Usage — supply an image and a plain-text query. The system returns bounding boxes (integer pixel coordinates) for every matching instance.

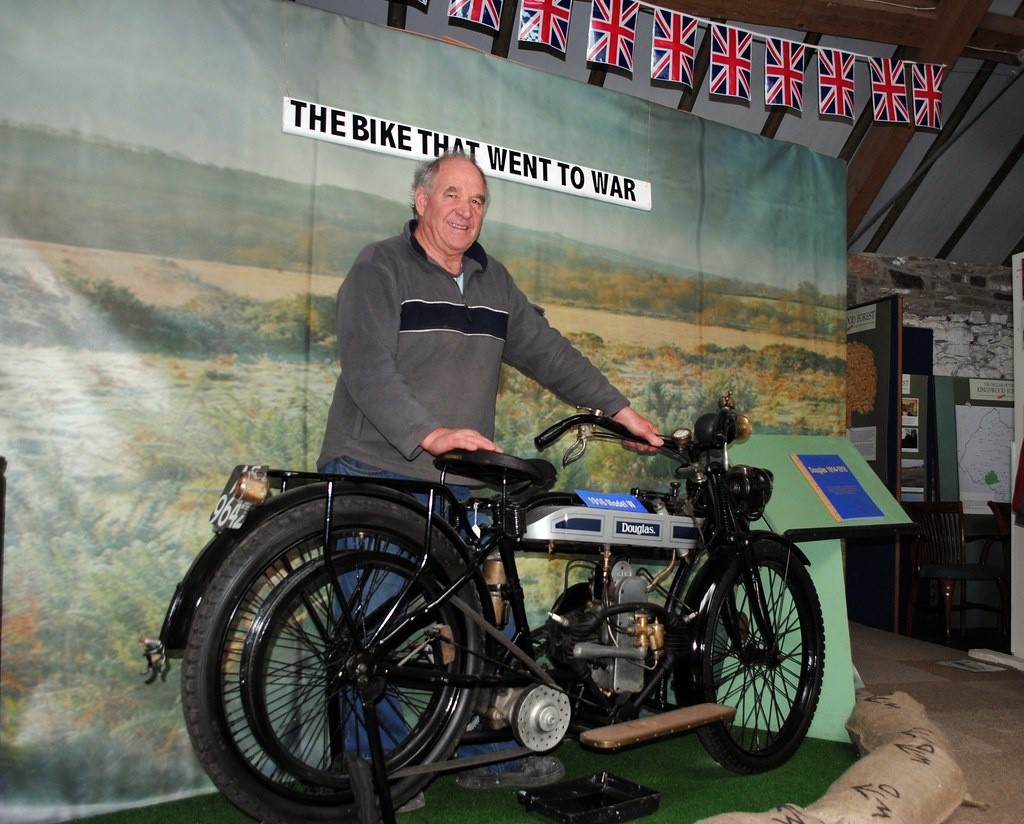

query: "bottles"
[483,549,509,626]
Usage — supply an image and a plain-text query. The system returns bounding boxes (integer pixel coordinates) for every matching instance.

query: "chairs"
[899,500,1007,647]
[988,501,1011,656]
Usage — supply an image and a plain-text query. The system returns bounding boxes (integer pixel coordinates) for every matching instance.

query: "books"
[790,454,885,523]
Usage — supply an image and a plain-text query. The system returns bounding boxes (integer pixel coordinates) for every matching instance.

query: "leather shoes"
[376,789,426,812]
[458,752,566,789]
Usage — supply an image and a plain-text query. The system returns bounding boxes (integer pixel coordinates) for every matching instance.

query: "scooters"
[139,379,828,824]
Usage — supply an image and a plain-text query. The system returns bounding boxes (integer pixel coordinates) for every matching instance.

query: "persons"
[315,153,664,814]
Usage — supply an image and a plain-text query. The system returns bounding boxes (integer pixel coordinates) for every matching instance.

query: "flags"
[709,23,752,103]
[764,37,805,113]
[817,47,856,120]
[650,8,697,89]
[868,56,910,123]
[912,63,946,130]
[517,0,573,53]
[586,0,640,72]
